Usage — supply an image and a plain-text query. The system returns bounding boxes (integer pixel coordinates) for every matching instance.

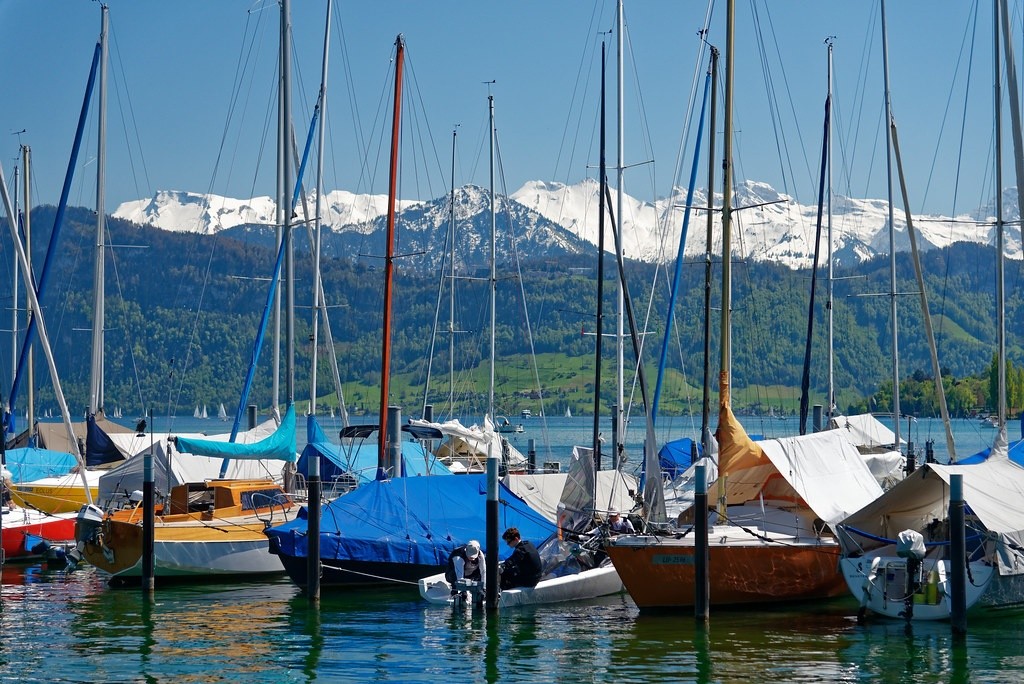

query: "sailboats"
[587,0,903,620]
[840,0,1022,617]
[0,0,538,587]
[262,30,692,615]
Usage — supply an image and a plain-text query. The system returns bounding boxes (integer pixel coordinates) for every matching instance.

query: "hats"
[608,507,620,516]
[466,540,481,559]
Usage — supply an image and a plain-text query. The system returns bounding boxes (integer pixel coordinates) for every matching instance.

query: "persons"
[500,527,542,588]
[444,539,486,595]
[584,506,635,568]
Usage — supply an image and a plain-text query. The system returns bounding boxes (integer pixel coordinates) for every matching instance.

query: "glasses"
[507,538,514,545]
